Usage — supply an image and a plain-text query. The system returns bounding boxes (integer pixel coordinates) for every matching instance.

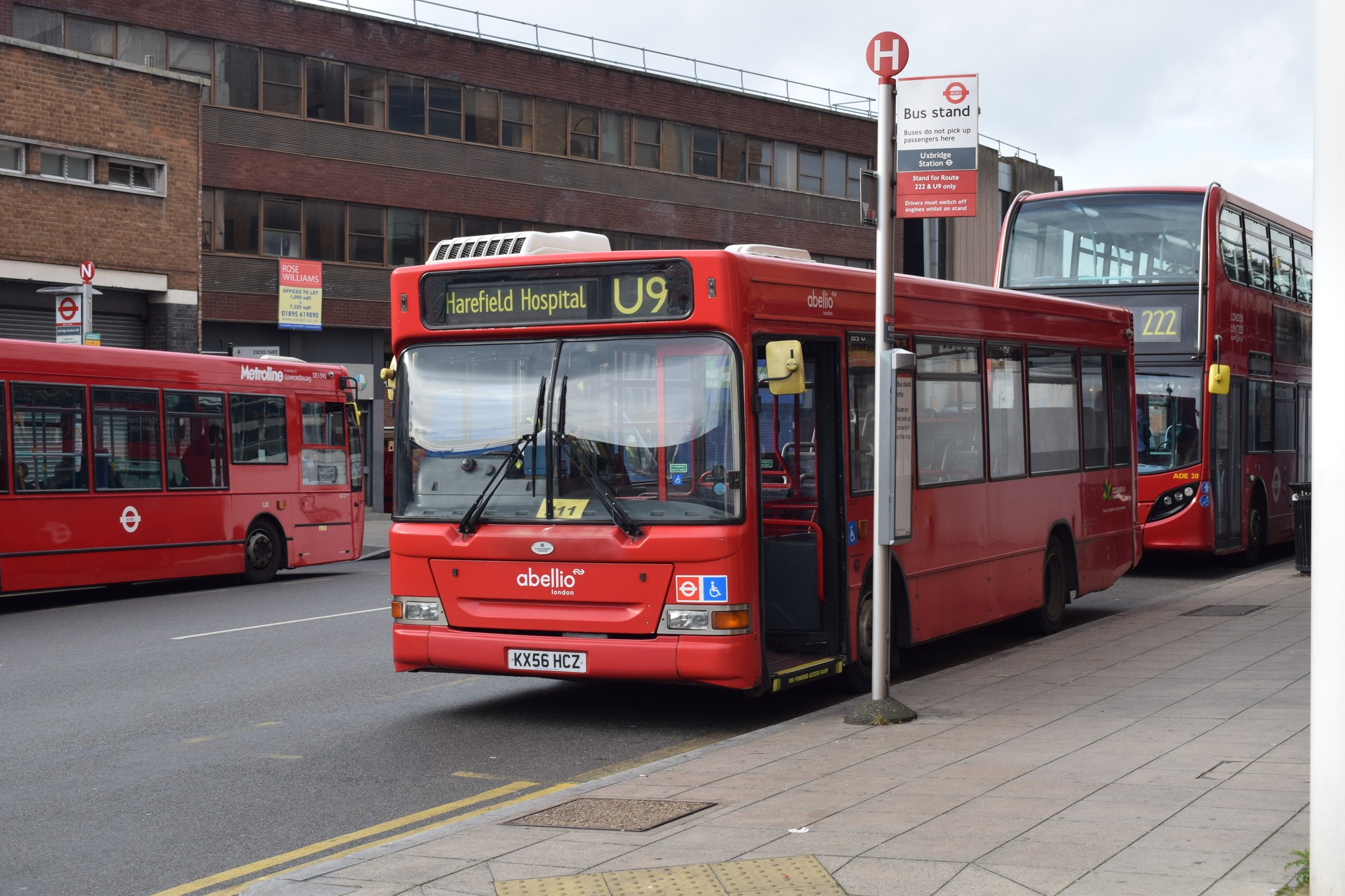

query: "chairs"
[1148,423,1197,467]
[1031,260,1313,304]
[682,404,1106,518]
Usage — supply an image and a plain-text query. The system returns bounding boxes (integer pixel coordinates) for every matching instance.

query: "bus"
[0,183,1312,717]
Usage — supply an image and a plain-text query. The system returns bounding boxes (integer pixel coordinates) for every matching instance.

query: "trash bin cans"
[1287,481,1312,577]
